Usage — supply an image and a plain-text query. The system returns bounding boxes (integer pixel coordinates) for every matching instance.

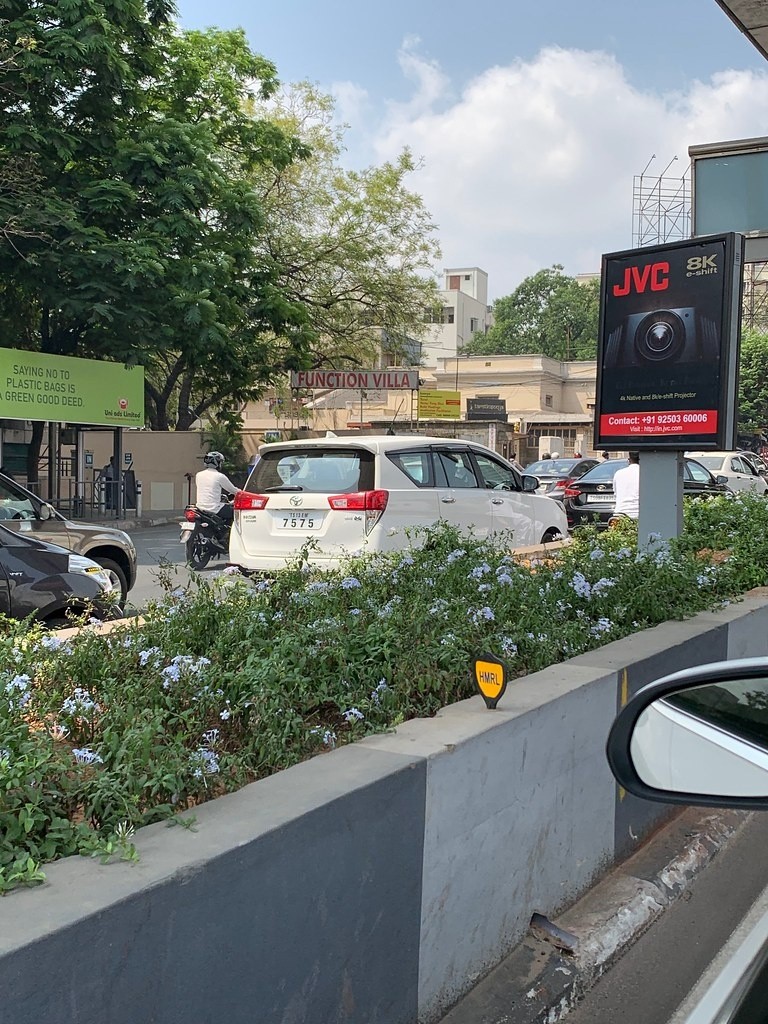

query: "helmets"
[203,452,225,471]
[543,453,550,460]
[550,451,560,460]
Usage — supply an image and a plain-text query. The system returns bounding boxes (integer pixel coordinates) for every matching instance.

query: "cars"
[561,456,736,541]
[683,449,768,503]
[731,449,768,488]
[0,522,115,637]
[517,458,602,527]
[403,459,513,491]
[603,655,768,1024]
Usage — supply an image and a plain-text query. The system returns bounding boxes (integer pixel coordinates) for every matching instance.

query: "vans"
[229,430,569,581]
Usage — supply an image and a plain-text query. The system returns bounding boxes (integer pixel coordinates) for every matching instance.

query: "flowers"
[0,491,768,891]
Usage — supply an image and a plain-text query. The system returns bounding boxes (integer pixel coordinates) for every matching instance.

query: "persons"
[508,453,526,473]
[542,453,551,460]
[195,452,243,553]
[550,452,559,460]
[0,466,16,482]
[612,450,640,523]
[602,452,610,459]
[435,454,468,487]
[573,452,582,458]
[101,456,116,510]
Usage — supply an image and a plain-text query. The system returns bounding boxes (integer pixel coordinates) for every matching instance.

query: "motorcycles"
[178,492,239,572]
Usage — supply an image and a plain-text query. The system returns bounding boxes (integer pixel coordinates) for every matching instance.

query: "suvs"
[0,473,137,612]
